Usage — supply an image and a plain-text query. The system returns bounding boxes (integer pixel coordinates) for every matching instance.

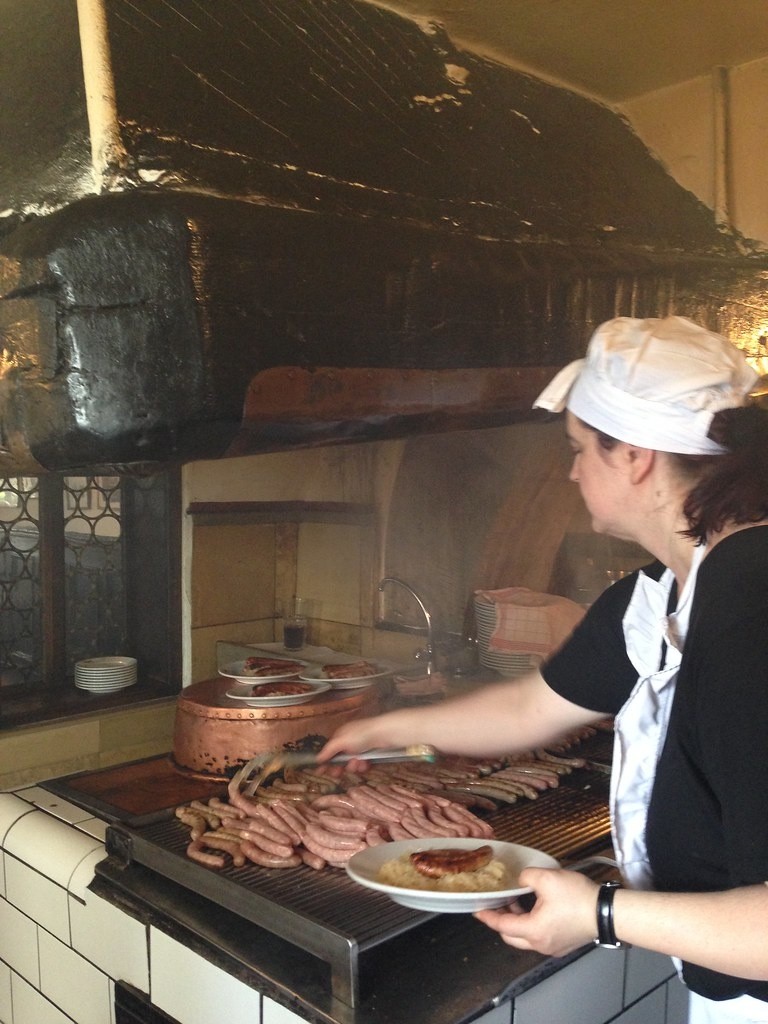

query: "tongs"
[267,743,440,770]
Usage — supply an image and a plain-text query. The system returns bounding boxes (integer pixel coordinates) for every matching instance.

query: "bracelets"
[592,879,630,954]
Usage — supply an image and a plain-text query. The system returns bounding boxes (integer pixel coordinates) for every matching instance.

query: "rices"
[381,857,506,891]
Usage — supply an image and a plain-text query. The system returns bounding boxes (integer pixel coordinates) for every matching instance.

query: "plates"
[299,667,394,689]
[474,595,536,678]
[74,656,136,693]
[226,681,332,708]
[346,837,561,913]
[218,660,302,684]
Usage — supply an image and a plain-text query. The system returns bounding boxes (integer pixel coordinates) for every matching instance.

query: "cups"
[282,598,309,651]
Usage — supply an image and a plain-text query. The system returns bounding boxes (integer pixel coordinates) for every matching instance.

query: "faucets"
[377,577,439,674]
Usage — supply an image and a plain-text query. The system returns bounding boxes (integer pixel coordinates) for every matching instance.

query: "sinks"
[373,666,494,708]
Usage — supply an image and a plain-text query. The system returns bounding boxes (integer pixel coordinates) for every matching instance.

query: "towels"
[473,587,593,667]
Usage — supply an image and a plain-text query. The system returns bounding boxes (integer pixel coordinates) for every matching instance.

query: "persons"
[322,316,768,1024]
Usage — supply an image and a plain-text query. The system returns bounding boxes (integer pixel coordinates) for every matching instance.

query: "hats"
[533,311,763,459]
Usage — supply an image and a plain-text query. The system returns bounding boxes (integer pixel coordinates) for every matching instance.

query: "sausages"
[253,681,310,694]
[177,714,613,871]
[244,656,304,676]
[323,661,376,677]
